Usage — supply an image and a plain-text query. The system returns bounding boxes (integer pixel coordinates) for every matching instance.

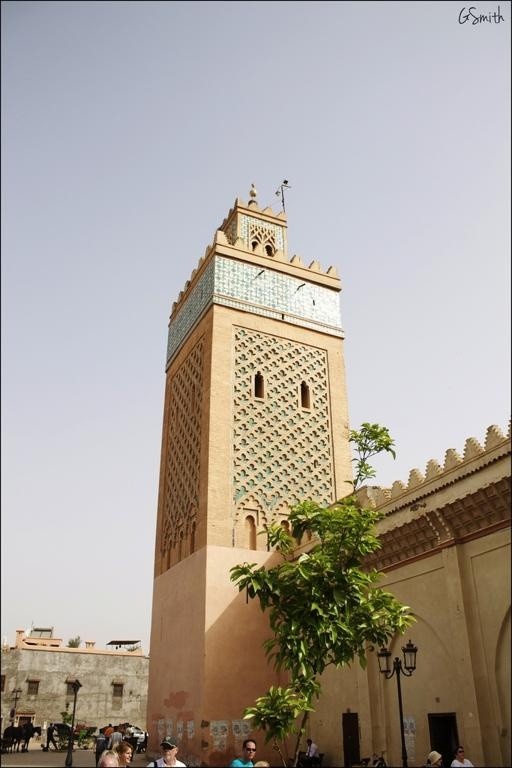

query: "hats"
[160,736,177,748]
[427,751,442,766]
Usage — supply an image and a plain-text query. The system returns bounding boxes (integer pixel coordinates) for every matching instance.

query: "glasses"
[457,750,465,753]
[162,745,173,750]
[245,745,256,752]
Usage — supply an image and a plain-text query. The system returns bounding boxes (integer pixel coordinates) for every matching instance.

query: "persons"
[228,739,256,767]
[146,735,187,767]
[73,719,149,767]
[46,722,58,751]
[424,750,443,768]
[253,759,269,767]
[302,738,321,766]
[450,746,474,767]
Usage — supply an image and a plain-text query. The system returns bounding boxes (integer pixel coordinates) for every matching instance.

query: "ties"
[308,745,310,757]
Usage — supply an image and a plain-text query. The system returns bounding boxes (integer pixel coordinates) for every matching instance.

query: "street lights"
[376,639,418,767]
[65,680,82,766]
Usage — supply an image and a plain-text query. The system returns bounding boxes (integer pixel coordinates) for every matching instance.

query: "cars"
[126,727,148,752]
